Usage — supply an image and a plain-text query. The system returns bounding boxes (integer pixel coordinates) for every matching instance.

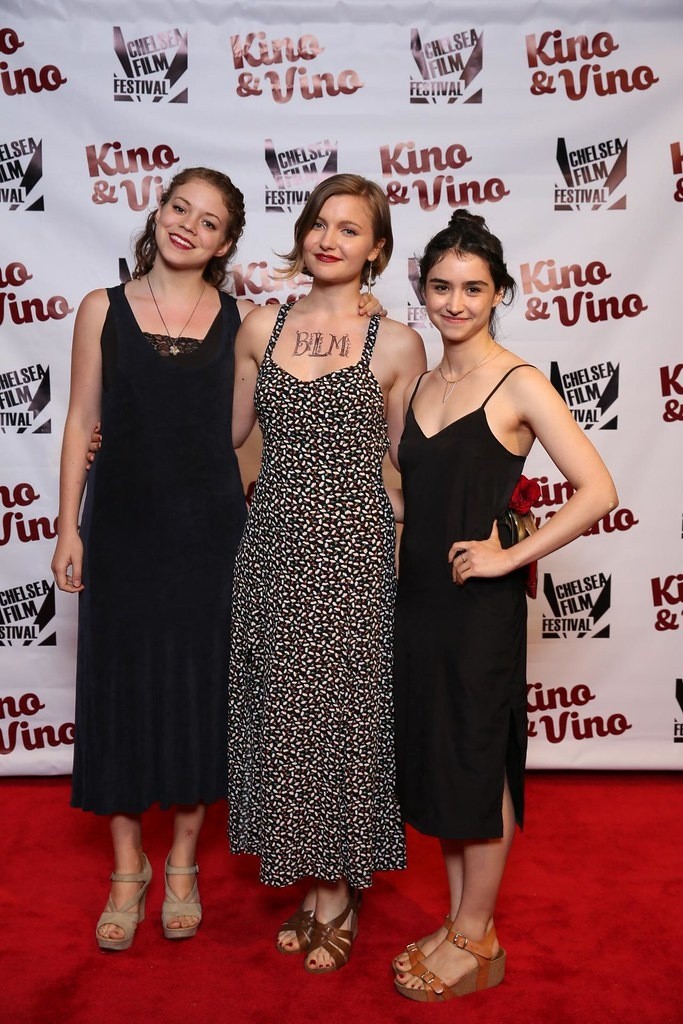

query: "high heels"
[161,848,202,939]
[275,896,316,954]
[304,888,363,974]
[394,921,506,1003]
[392,913,456,973]
[95,853,152,951]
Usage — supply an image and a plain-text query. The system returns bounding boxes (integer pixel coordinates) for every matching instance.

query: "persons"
[51,168,386,950]
[85,174,428,973]
[244,210,619,1002]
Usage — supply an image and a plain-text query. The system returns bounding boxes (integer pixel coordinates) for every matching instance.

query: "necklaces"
[438,342,508,403]
[145,275,206,355]
